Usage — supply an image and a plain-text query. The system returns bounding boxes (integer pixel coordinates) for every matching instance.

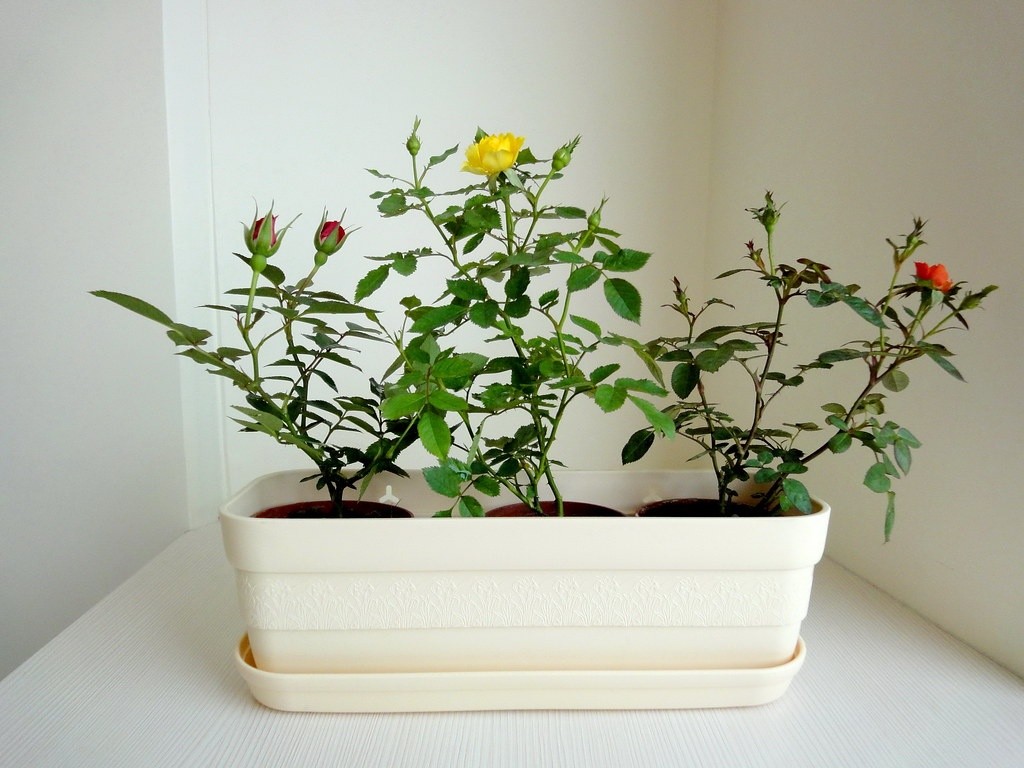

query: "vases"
[217,467,832,714]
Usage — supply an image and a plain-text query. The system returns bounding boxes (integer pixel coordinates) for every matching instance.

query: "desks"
[0,520,1024,768]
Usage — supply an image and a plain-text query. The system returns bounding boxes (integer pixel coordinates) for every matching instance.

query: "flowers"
[87,113,998,546]
